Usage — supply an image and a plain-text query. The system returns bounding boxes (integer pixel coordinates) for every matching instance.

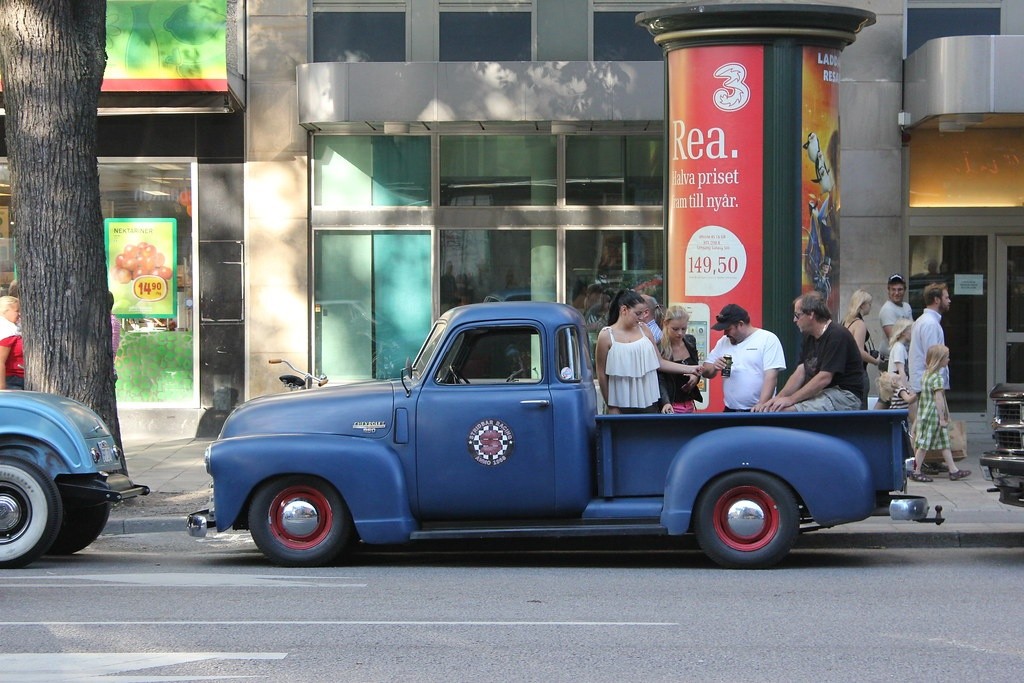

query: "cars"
[0,389,150,569]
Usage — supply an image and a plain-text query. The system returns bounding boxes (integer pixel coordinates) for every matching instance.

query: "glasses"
[716,315,739,323]
[794,311,807,319]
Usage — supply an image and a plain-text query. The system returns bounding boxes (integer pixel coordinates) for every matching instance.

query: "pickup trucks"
[187,301,945,568]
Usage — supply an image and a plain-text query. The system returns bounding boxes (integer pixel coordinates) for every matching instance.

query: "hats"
[711,304,748,331]
[887,273,906,285]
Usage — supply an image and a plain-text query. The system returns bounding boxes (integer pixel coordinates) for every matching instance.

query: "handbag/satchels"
[909,391,968,463]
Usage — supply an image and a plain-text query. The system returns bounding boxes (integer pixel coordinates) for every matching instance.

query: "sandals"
[911,473,934,482]
[950,469,972,481]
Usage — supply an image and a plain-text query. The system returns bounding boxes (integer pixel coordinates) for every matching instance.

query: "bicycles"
[268,358,329,392]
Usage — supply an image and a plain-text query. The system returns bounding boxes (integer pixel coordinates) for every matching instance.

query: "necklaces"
[820,324,826,335]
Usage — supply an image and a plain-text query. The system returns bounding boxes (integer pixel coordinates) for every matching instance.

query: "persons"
[759,295,864,412]
[0,279,24,390]
[909,284,951,471]
[877,372,938,475]
[640,295,663,342]
[910,345,971,481]
[702,304,786,412]
[655,306,703,414]
[839,290,885,410]
[874,274,913,409]
[584,284,609,361]
[595,289,704,414]
[109,292,121,381]
[888,319,913,376]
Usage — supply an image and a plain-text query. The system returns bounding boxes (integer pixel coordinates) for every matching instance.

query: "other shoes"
[932,461,949,472]
[920,463,938,474]
